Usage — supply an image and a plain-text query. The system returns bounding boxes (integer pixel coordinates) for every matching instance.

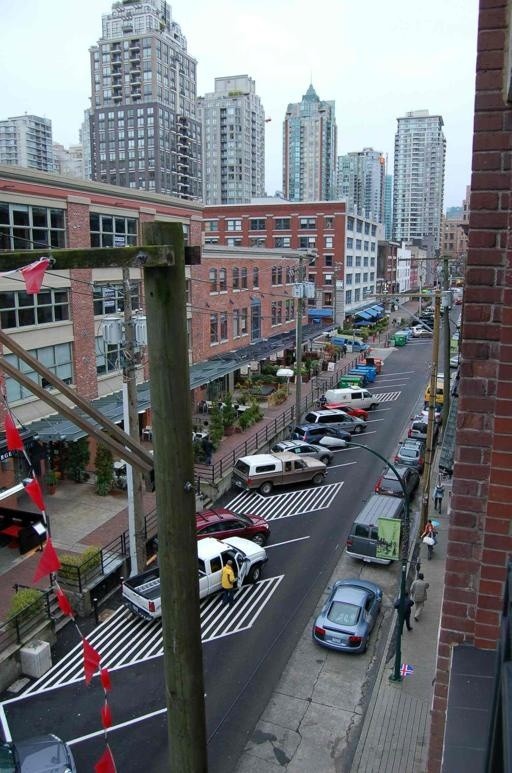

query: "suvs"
[304,408,366,436]
[292,423,352,444]
[395,437,425,474]
[407,414,440,443]
[197,509,271,547]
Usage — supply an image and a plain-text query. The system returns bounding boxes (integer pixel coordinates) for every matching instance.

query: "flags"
[400,663,415,678]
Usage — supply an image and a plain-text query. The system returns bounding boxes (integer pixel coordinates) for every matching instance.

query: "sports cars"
[313,577,382,655]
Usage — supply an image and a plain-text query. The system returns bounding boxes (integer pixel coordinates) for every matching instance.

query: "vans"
[325,387,379,411]
[424,383,445,406]
[345,492,408,567]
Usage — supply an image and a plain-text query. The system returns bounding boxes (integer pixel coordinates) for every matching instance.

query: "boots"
[427,550,432,560]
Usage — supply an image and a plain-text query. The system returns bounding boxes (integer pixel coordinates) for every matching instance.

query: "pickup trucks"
[121,535,269,624]
[230,451,328,495]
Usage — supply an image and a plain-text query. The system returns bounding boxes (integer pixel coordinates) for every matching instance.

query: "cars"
[420,403,443,423]
[270,440,334,464]
[0,734,76,773]
[377,462,420,502]
[413,330,432,338]
[450,356,459,369]
[322,405,368,421]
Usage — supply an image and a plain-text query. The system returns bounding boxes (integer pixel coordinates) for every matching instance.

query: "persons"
[394,591,414,632]
[410,573,429,623]
[221,560,238,603]
[422,519,436,560]
[432,479,445,514]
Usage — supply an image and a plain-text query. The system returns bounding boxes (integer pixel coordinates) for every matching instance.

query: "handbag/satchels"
[436,485,445,492]
[413,592,427,602]
[422,531,435,546]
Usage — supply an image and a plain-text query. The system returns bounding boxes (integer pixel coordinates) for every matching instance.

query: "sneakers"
[407,626,414,632]
[229,601,236,609]
[223,599,230,605]
[438,511,441,514]
[434,507,437,510]
[413,616,419,622]
